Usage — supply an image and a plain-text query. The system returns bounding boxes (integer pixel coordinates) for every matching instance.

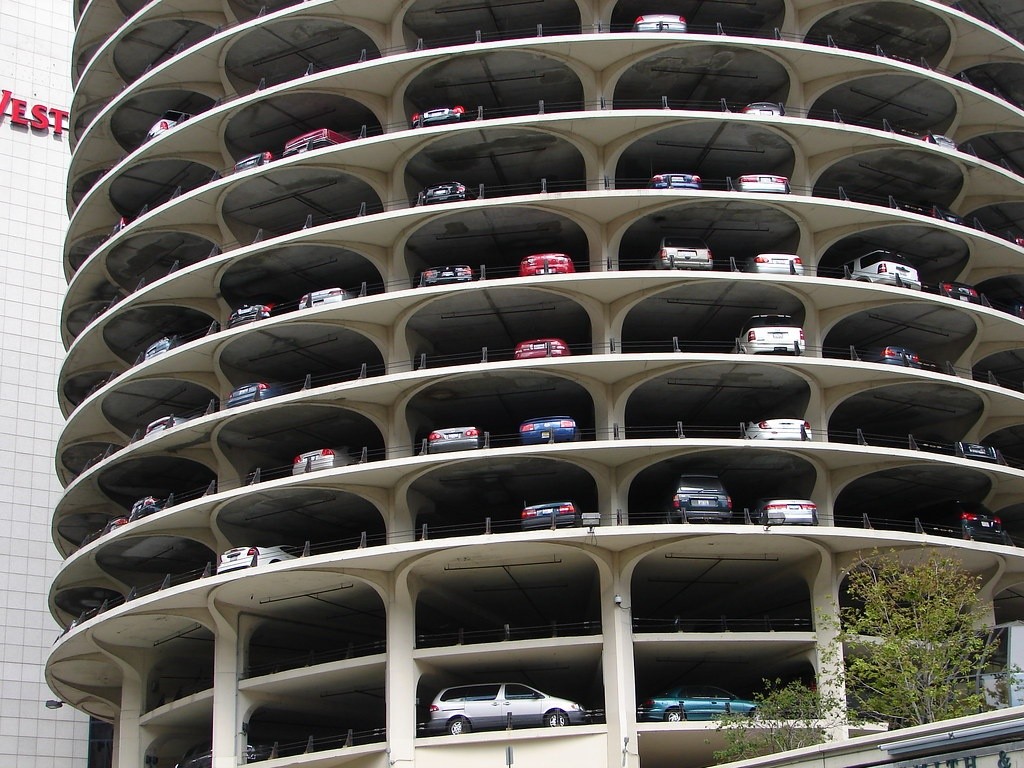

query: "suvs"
[429,682,587,735]
[663,473,732,526]
[929,495,1004,544]
[732,314,806,356]
[827,250,921,292]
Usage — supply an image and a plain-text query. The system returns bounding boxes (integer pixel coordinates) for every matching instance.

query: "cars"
[146,109,198,141]
[514,339,571,360]
[744,417,812,440]
[428,425,480,453]
[877,345,920,368]
[742,102,782,116]
[234,128,354,176]
[935,282,980,305]
[143,416,193,437]
[228,303,278,330]
[145,336,190,360]
[648,173,702,190]
[518,415,580,444]
[645,683,769,722]
[101,496,166,538]
[298,288,361,311]
[522,498,583,529]
[913,201,963,226]
[751,495,820,526]
[633,15,686,33]
[957,441,997,464]
[736,173,791,193]
[412,106,465,128]
[420,265,471,287]
[749,253,805,275]
[227,380,290,409]
[919,133,956,151]
[292,448,363,476]
[216,543,305,576]
[519,252,577,277]
[421,182,478,205]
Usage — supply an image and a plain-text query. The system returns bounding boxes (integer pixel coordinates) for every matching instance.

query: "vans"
[639,236,714,270]
